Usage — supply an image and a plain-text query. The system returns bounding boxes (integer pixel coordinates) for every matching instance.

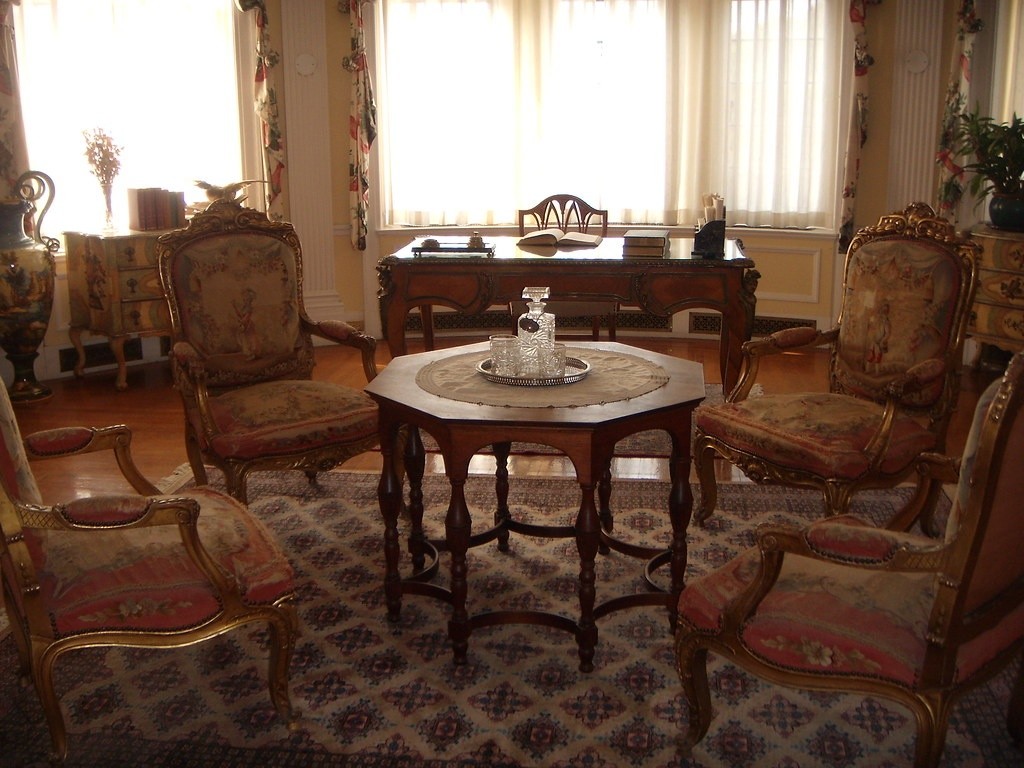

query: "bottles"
[517,287,556,374]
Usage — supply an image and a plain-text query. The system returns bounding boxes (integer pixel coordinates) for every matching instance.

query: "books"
[127,187,185,231]
[516,227,603,247]
[622,229,671,257]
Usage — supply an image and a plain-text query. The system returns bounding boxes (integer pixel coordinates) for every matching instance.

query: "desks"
[373,235,761,399]
[365,341,707,671]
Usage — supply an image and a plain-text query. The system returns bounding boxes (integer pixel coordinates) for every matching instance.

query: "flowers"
[84,129,123,208]
[937,100,1024,217]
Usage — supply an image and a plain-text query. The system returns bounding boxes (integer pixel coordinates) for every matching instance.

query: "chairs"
[156,200,405,514]
[510,196,618,343]
[671,355,1023,764]
[693,202,986,525]
[2,376,305,762]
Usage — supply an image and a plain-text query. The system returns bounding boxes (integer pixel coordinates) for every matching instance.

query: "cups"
[489,334,519,377]
[537,342,566,377]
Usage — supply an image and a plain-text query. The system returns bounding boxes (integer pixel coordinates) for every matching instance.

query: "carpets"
[0,462,1021,765]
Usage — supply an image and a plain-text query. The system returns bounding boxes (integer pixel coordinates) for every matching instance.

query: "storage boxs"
[690,205,728,259]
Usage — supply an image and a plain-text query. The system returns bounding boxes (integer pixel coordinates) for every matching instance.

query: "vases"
[989,191,1024,232]
[100,180,115,235]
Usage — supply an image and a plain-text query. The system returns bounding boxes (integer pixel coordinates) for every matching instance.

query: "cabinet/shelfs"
[953,223,1022,377]
[61,222,184,384]
